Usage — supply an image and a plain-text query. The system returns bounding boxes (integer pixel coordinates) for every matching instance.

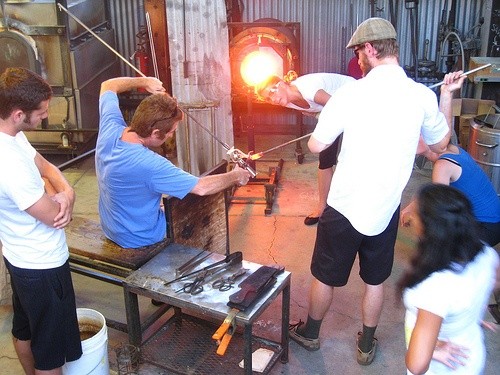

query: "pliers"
[238,150,254,169]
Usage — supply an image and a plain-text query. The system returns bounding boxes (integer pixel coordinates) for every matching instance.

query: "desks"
[122,242,291,375]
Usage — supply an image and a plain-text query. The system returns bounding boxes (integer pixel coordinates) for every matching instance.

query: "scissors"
[183,269,209,295]
[212,274,235,292]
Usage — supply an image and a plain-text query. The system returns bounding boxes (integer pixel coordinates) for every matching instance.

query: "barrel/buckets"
[469,114,500,196]
[59,307,110,375]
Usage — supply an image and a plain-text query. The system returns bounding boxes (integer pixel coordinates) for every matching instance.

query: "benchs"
[65,215,181,363]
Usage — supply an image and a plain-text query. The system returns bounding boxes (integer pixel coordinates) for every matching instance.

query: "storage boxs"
[467,57,500,83]
[452,98,496,149]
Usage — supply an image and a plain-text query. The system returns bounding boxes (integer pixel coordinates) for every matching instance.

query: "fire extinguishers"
[133,43,149,93]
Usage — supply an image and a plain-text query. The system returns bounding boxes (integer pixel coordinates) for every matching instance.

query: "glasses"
[265,82,280,103]
[353,43,373,59]
[151,104,178,127]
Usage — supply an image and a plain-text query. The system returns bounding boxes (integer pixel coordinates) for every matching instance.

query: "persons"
[403,184,499,375]
[289,17,467,367]
[1,67,84,374]
[256,73,358,225]
[401,130,500,327]
[95,75,251,248]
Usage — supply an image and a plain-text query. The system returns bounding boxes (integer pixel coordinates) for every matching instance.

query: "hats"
[346,18,397,49]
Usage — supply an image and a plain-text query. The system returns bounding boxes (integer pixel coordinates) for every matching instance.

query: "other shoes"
[304,215,319,225]
[288,318,320,351]
[357,332,378,365]
